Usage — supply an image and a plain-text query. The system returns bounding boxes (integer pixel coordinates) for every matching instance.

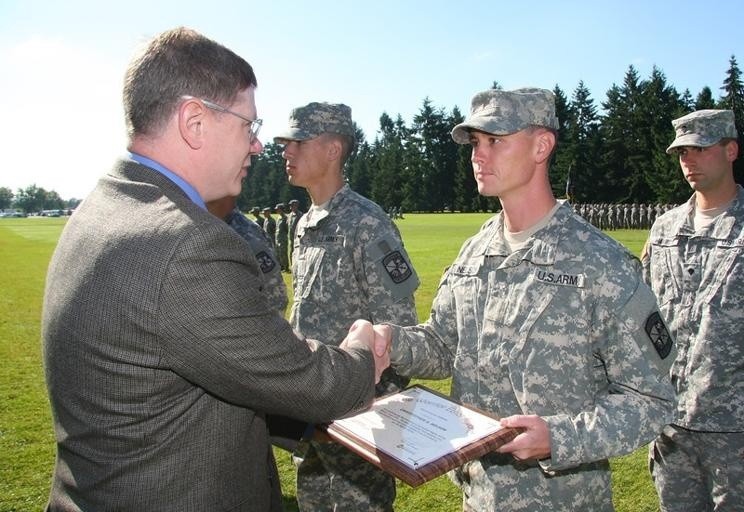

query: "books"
[321,384,523,488]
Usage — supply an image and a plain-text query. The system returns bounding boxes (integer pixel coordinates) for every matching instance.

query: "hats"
[272,101,356,146]
[664,108,739,156]
[451,86,560,145]
[247,198,300,215]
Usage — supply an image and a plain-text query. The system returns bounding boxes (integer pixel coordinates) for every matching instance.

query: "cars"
[0,207,74,217]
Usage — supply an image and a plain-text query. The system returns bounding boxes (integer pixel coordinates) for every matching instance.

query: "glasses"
[179,94,264,144]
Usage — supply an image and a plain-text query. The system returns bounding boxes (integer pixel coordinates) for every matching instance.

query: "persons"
[340,87,679,512]
[398,206,404,219]
[205,199,303,321]
[44,24,391,512]
[389,207,393,218]
[641,109,744,512]
[393,206,397,219]
[272,101,420,512]
[570,201,679,231]
